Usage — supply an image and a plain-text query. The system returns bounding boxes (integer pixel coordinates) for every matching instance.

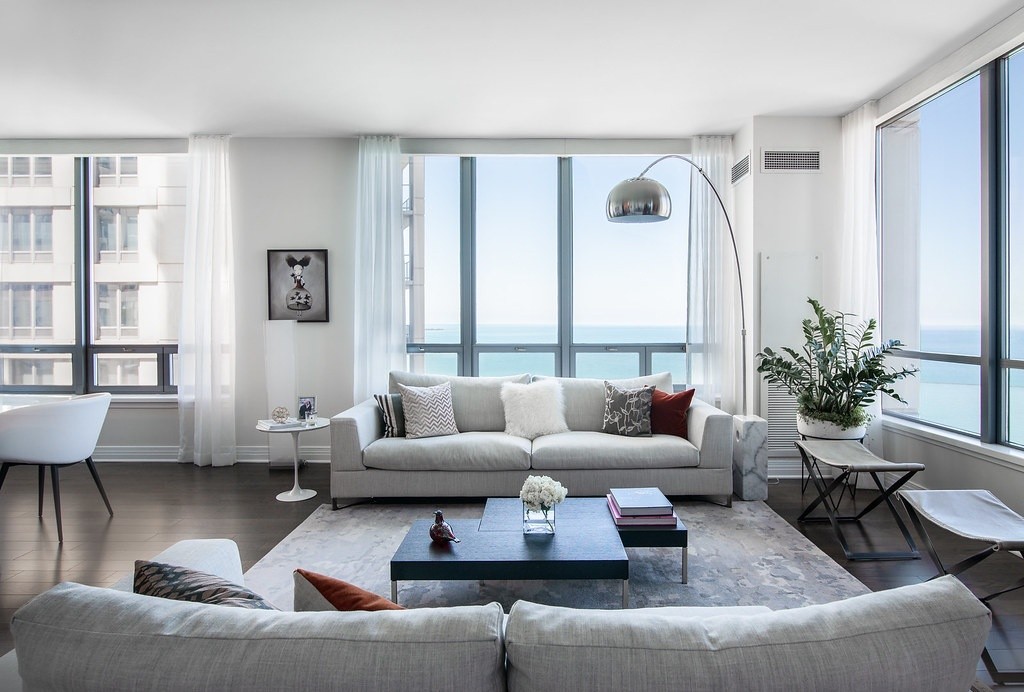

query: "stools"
[791,439,927,562]
[894,487,1024,687]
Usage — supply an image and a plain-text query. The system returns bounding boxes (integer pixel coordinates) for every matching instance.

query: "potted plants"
[756,294,920,440]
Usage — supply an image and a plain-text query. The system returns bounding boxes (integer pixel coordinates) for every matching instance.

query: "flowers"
[519,475,568,533]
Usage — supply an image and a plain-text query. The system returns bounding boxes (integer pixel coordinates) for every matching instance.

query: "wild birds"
[429,510,462,544]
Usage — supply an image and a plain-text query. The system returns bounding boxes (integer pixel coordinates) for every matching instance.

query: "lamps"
[606,154,770,502]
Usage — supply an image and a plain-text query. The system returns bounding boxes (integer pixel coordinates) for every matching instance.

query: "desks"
[795,428,865,511]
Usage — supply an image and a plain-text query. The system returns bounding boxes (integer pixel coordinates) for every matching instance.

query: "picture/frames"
[266,249,330,323]
[298,396,317,422]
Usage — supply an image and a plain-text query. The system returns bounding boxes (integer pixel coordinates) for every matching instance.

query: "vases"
[523,501,556,536]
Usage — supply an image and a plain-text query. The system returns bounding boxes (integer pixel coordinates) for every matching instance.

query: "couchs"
[13,537,992,692]
[329,370,736,513]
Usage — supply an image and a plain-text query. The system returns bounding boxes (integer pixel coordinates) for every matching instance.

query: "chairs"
[0,391,115,544]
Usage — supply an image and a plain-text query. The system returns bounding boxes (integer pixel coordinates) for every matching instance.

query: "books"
[605,487,677,526]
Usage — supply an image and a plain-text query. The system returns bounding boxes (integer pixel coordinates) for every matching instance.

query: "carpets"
[242,502,875,615]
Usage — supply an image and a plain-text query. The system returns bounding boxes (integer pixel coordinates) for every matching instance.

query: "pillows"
[602,379,657,438]
[290,567,404,613]
[644,383,696,441]
[499,378,571,443]
[373,392,405,437]
[396,380,461,441]
[133,557,284,612]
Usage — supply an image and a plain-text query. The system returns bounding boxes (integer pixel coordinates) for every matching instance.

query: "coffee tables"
[390,517,630,610]
[479,498,690,584]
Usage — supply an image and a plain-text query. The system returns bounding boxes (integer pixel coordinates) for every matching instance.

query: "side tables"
[256,416,331,502]
